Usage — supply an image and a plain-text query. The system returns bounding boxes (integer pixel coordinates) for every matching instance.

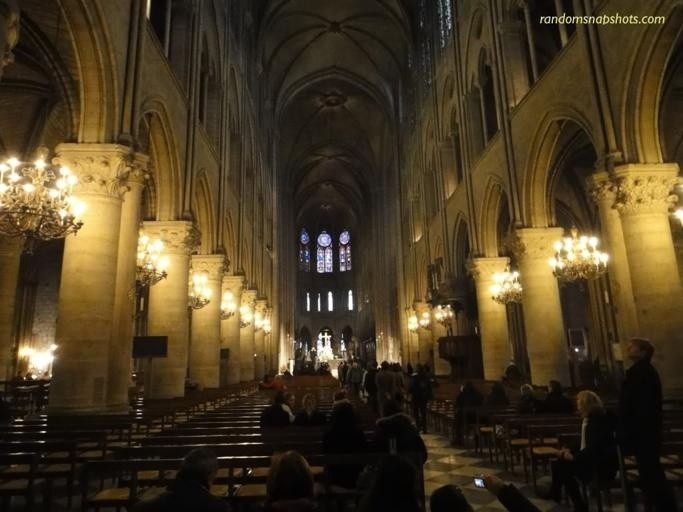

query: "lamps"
[135,232,273,336]
[0,0,88,257]
[487,268,525,306]
[408,305,455,335]
[547,225,611,285]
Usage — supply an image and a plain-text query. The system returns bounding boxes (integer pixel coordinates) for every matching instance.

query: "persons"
[614,336,677,512]
[134,447,232,512]
[254,354,618,512]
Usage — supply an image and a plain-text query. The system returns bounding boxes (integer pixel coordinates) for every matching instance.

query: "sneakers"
[534,487,562,503]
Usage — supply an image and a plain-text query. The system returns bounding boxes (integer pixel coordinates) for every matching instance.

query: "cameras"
[473,473,486,488]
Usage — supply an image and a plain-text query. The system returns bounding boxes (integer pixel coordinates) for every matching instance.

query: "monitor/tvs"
[133,336,168,357]
